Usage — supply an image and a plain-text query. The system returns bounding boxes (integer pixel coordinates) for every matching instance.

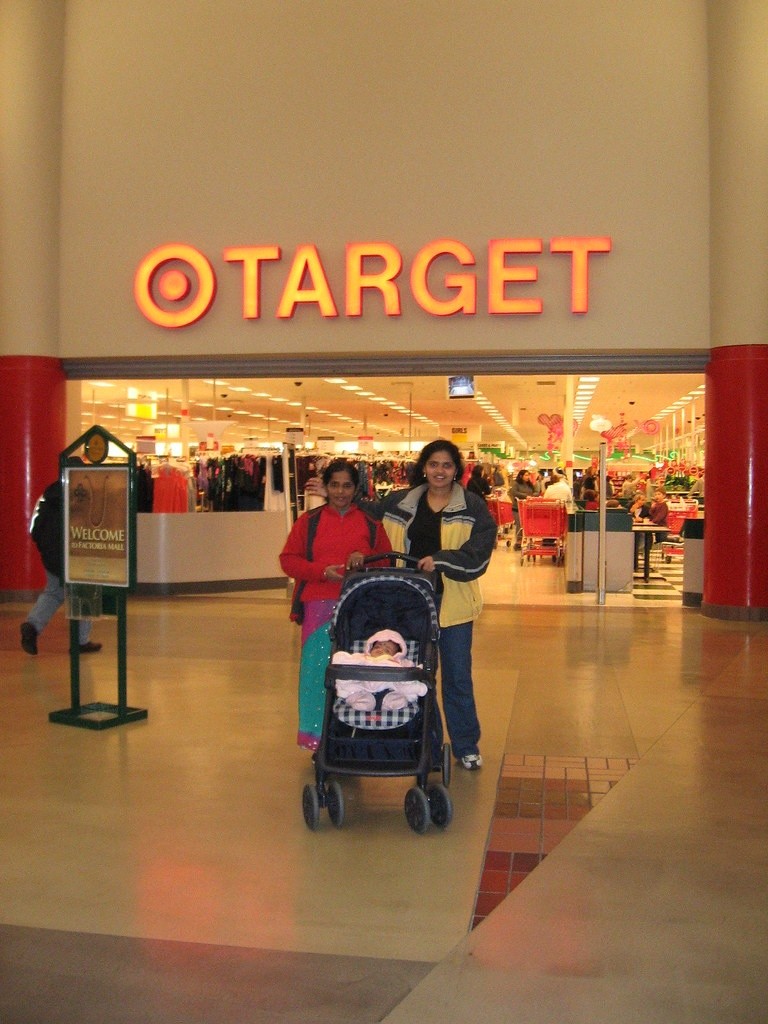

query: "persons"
[507,466,704,551]
[493,464,504,487]
[466,465,492,506]
[331,629,427,712]
[306,439,498,770]
[278,461,397,752]
[19,455,103,655]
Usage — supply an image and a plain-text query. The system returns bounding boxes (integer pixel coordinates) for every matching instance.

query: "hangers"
[139,456,192,476]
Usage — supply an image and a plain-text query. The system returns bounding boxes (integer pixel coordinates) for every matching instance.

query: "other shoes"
[20,623,38,656]
[71,642,102,653]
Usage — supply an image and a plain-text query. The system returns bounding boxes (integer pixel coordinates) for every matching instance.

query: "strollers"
[300,550,453,834]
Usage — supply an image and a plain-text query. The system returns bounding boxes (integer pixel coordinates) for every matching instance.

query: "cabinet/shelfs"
[610,476,624,494]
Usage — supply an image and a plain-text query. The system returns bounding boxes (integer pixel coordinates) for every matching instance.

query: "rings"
[356,562,360,567]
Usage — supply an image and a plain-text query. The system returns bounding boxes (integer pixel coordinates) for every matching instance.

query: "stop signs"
[690,467,697,475]
[667,468,675,475]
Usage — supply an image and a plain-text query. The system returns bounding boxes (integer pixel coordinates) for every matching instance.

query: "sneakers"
[462,754,482,769]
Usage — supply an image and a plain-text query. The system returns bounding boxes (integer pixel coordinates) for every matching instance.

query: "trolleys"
[515,495,568,566]
[660,498,700,564]
[487,492,514,548]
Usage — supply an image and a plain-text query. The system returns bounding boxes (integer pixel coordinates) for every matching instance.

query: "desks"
[632,520,657,573]
[632,525,672,584]
[563,498,634,593]
